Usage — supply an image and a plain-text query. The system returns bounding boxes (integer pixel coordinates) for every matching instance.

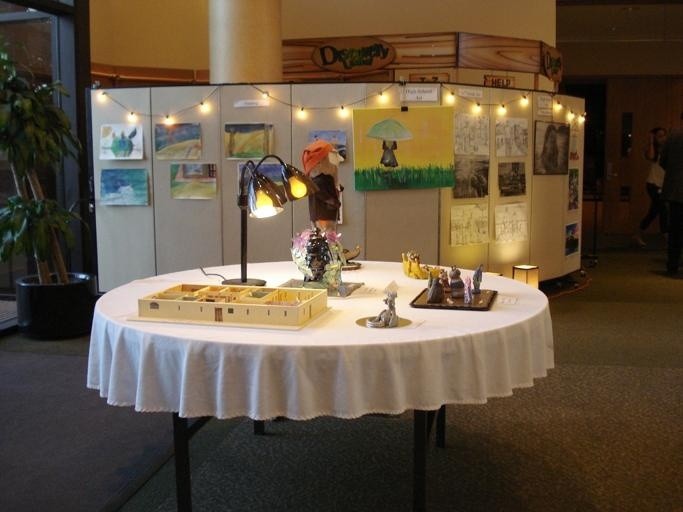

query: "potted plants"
[1,44,91,341]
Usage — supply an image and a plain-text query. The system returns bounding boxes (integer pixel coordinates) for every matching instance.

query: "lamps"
[513,265,539,289]
[222,153,321,286]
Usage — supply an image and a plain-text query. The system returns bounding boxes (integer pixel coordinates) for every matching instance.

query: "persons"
[631,126,669,249]
[539,124,559,172]
[657,110,682,277]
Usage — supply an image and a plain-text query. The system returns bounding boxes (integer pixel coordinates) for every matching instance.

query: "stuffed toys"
[299,138,345,270]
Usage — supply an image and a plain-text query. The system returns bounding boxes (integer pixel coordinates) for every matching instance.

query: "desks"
[92,260,548,507]
